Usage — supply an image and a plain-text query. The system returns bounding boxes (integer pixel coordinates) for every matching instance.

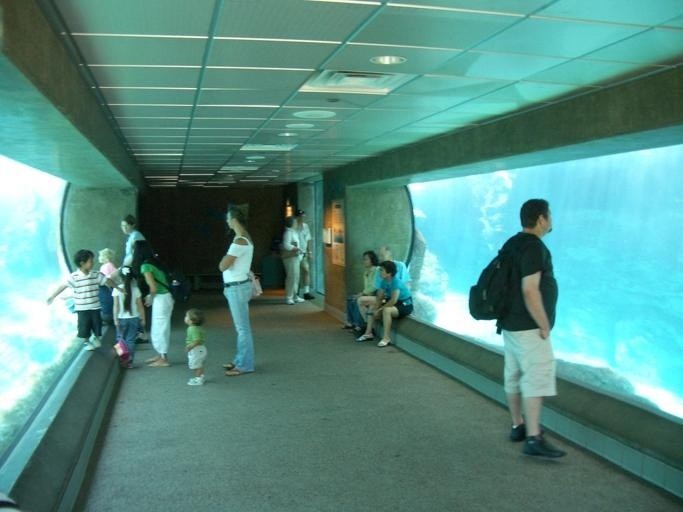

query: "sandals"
[349,326,361,336]
[147,360,172,367]
[342,323,355,330]
[222,362,235,369]
[144,357,165,364]
[225,367,248,377]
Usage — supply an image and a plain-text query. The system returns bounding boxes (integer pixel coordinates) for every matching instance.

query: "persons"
[280,216,306,305]
[341,250,378,332]
[47,203,255,386]
[352,246,413,337]
[296,209,315,299]
[497,199,568,461]
[356,261,413,347]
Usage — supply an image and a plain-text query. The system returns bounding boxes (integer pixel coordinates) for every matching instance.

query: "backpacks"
[151,250,192,303]
[468,246,518,322]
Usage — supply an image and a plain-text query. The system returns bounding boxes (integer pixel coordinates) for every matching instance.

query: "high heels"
[355,333,374,342]
[376,337,392,347]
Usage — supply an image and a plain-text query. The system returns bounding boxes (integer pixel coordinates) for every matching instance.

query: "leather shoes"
[222,278,254,287]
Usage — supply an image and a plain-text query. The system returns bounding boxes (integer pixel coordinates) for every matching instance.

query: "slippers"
[136,335,149,344]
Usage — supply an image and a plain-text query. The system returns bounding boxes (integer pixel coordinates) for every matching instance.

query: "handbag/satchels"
[278,242,299,260]
[113,338,130,360]
[248,270,264,299]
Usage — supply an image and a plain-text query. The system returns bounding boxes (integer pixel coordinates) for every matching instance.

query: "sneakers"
[189,375,207,382]
[294,297,305,303]
[303,293,315,300]
[83,341,96,351]
[521,439,568,461]
[510,423,545,442]
[286,299,295,305]
[91,338,102,349]
[186,376,205,386]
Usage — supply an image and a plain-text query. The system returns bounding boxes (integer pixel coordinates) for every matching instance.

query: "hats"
[294,209,307,217]
